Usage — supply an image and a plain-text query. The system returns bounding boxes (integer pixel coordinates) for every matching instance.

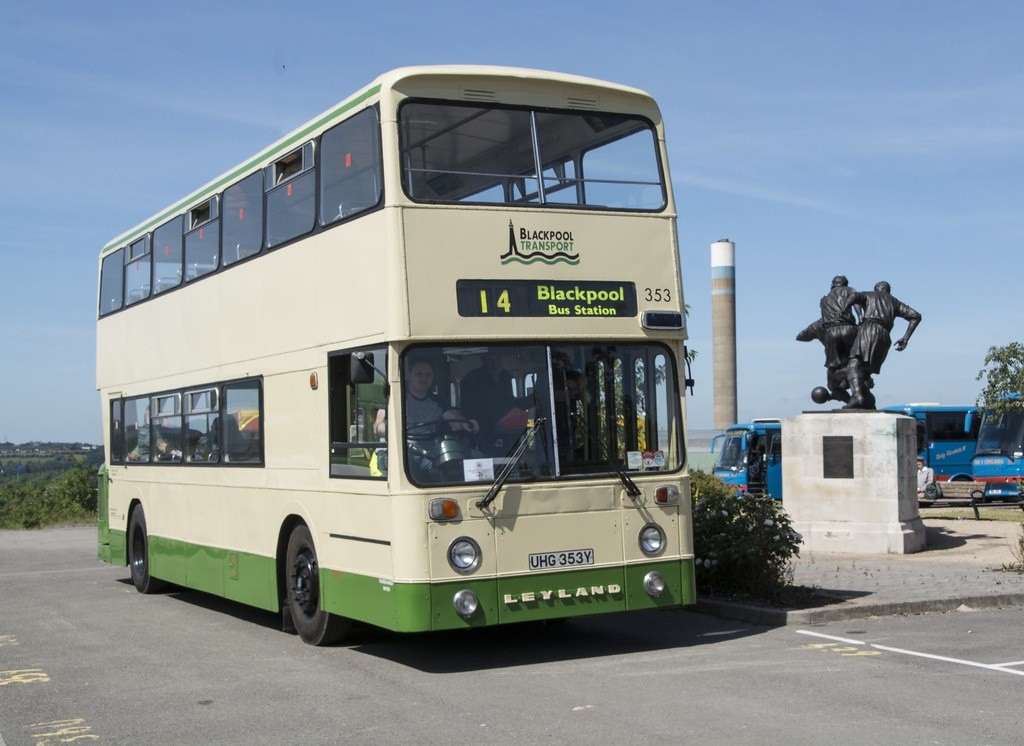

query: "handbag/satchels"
[924,483,943,500]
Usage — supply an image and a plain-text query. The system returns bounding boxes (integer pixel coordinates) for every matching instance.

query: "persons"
[126,405,219,461]
[796,275,921,409]
[916,457,934,499]
[372,356,479,484]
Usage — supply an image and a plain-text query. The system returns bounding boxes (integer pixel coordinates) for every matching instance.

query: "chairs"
[109,201,371,313]
[112,426,218,462]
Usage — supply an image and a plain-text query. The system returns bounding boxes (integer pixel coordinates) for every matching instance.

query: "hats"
[916,456,924,460]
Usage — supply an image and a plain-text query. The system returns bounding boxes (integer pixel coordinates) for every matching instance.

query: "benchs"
[918,481,987,521]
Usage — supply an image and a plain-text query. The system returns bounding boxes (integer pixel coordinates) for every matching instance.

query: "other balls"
[811,386,830,404]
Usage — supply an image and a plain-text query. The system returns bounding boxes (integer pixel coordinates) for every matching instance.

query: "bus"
[97,64,698,646]
[710,418,781,501]
[879,402,986,507]
[965,392,1024,512]
